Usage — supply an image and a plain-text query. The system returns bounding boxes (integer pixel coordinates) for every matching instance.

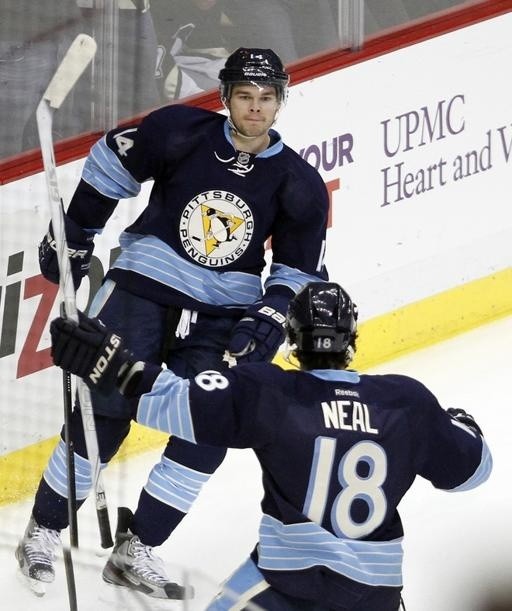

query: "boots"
[103,507,186,599]
[15,512,64,583]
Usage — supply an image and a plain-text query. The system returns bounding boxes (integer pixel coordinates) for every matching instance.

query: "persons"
[13,46,330,601]
[48,278,494,611]
[1,1,469,162]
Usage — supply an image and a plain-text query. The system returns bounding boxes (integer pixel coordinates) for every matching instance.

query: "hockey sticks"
[36,35,119,547]
[59,279,113,568]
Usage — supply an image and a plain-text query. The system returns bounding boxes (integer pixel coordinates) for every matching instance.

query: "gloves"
[39,198,95,291]
[49,302,135,398]
[228,283,297,365]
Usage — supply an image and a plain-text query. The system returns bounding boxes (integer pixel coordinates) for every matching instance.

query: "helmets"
[286,280,359,356]
[218,47,289,114]
